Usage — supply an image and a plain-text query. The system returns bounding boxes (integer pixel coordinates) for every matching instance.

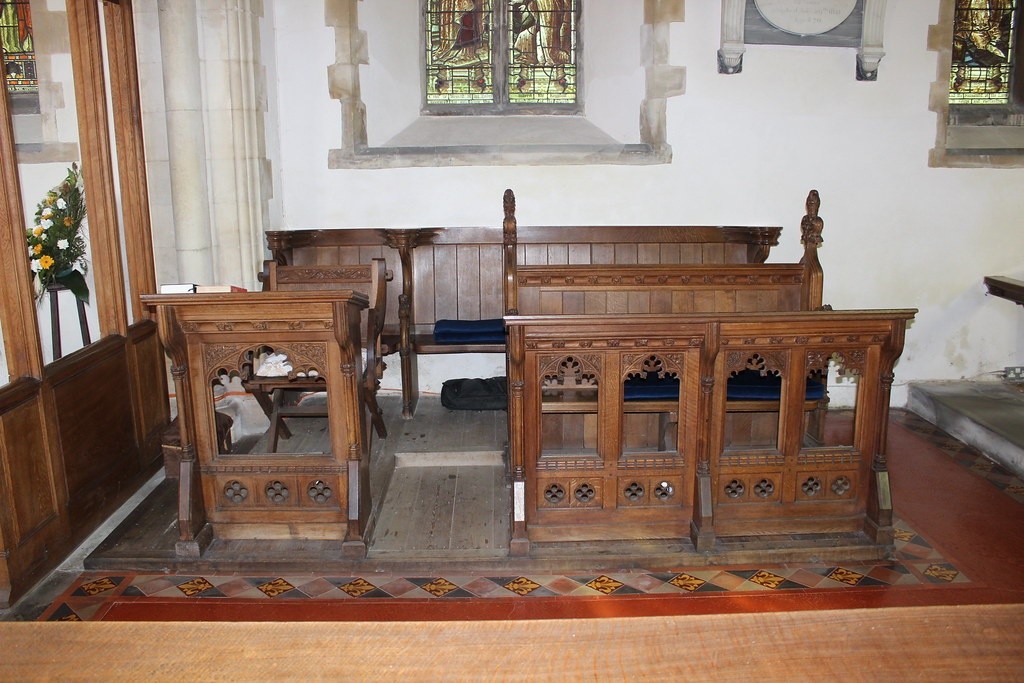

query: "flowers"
[25,163,93,305]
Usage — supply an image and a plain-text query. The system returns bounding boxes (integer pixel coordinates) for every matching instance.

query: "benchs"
[238,189,838,493]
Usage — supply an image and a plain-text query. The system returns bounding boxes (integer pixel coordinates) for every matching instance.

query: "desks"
[47,282,91,361]
